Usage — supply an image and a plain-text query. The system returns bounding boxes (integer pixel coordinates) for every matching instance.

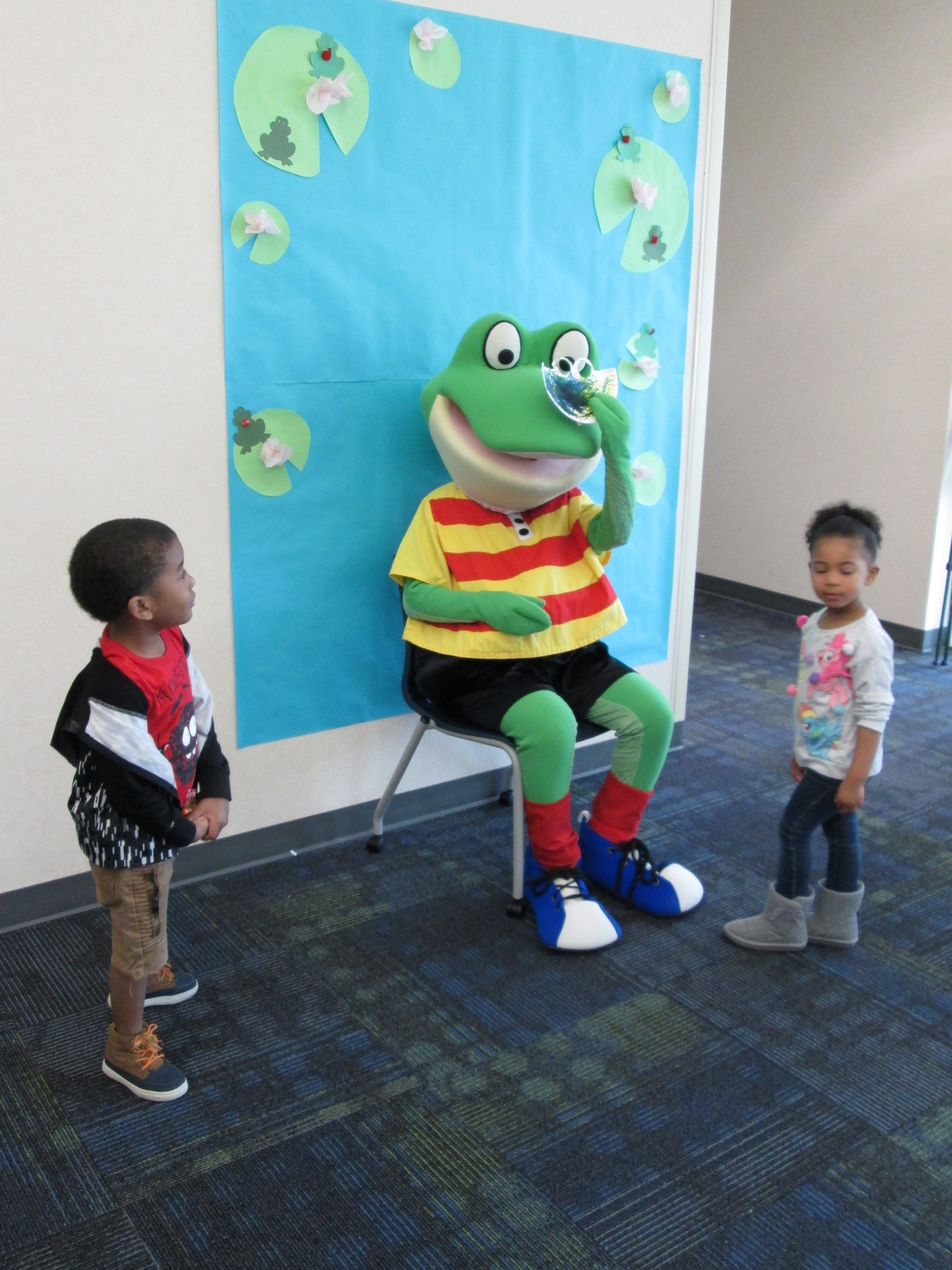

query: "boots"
[724,881,817,952]
[805,878,866,947]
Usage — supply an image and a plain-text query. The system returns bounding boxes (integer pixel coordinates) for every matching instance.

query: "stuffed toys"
[389,312,704,951]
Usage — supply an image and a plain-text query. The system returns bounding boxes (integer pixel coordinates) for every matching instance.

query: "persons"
[723,498,894,950]
[50,517,231,1101]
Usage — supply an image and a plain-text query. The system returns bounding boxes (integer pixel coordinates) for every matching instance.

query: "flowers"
[242,207,282,236]
[307,74,352,115]
[635,355,661,380]
[631,178,661,207]
[634,466,658,482]
[665,74,688,109]
[258,436,291,469]
[415,14,448,49]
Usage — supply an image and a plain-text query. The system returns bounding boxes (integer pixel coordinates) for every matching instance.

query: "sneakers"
[102,1021,188,1100]
[108,963,200,1010]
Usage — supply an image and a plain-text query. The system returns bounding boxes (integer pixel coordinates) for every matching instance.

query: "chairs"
[367,585,613,917]
